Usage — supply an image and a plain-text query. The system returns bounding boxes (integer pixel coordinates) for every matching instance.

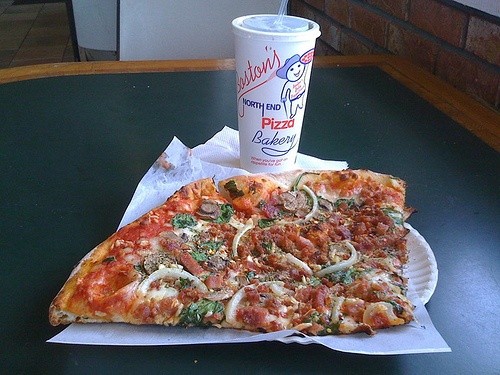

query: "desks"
[0,54,499,375]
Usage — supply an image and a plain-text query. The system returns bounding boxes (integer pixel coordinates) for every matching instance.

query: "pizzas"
[48,171,418,336]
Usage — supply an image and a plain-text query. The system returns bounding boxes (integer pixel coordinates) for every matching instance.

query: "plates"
[401,220,439,306]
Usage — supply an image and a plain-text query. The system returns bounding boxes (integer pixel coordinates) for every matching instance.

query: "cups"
[231,13,322,173]
